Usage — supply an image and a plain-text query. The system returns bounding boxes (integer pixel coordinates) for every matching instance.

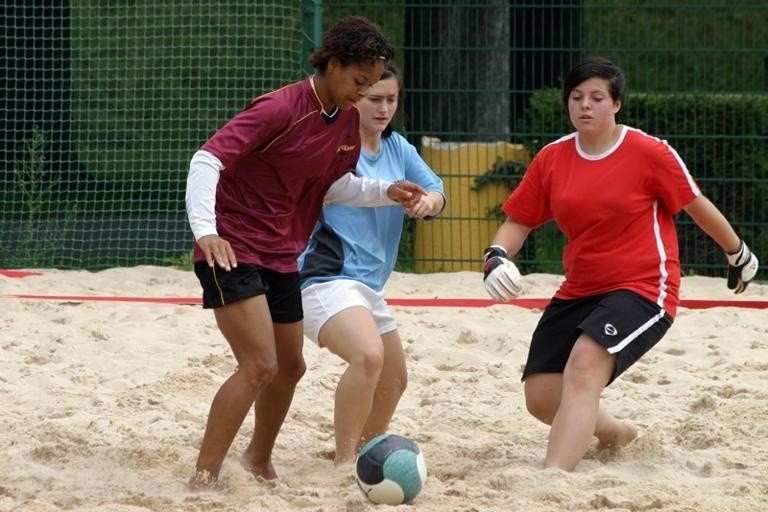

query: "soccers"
[356,434,425,505]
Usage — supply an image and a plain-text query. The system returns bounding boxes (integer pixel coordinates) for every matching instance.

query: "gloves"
[726,240,759,294]
[482,245,526,303]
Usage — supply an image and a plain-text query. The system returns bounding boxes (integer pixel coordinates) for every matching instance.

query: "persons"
[483,55,759,473]
[184,13,429,491]
[298,63,448,465]
